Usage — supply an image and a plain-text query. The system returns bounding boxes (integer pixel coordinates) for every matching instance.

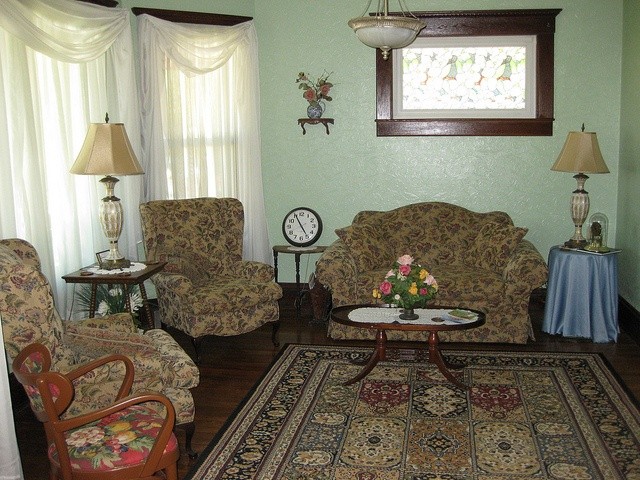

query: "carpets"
[184,343,640,480]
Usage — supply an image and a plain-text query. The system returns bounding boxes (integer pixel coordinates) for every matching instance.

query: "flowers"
[373,254,439,306]
[296,69,335,105]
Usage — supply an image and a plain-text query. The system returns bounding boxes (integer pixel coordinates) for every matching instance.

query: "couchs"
[314,201,548,345]
[140,198,282,364]
[1,238,199,478]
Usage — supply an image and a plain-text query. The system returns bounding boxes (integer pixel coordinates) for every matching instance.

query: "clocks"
[283,207,323,247]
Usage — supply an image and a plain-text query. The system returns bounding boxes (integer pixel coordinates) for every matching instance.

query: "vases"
[307,100,326,119]
[399,307,419,320]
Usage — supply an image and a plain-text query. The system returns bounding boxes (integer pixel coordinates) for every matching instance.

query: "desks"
[541,245,620,344]
[330,304,486,392]
[273,244,330,345]
[62,260,167,330]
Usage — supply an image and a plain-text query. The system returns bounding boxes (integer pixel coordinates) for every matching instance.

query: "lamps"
[73,111,145,271]
[347,1,426,59]
[549,123,610,249]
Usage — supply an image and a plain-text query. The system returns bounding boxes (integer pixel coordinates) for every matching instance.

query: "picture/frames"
[368,8,563,137]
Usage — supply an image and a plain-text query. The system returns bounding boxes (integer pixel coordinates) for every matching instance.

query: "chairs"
[11,342,179,479]
